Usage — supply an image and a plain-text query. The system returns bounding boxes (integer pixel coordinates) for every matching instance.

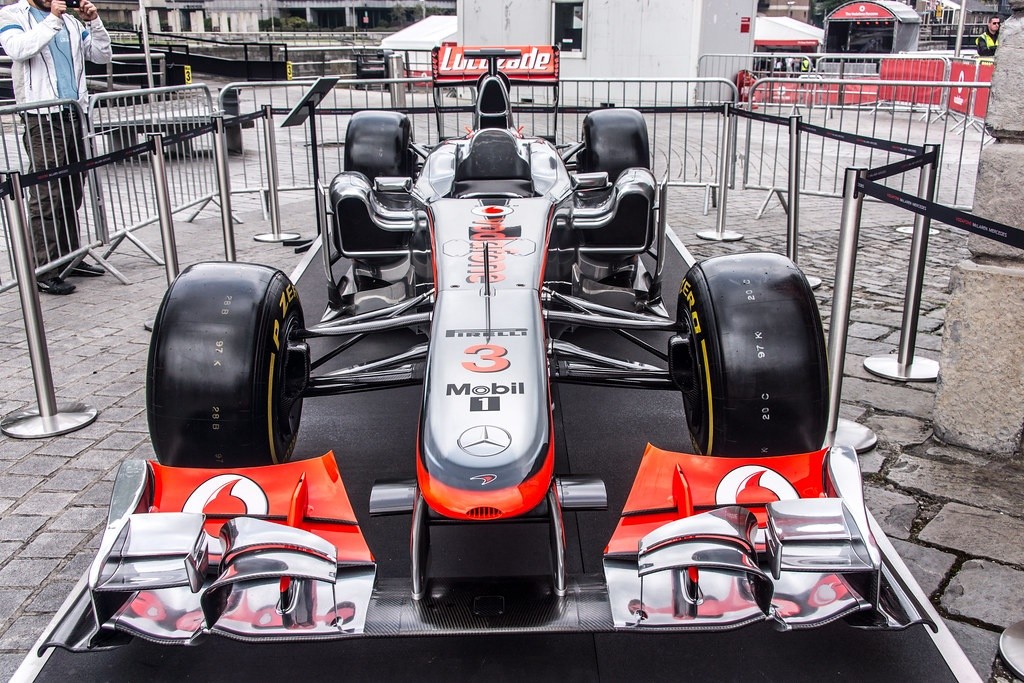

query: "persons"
[776,58,809,78]
[0,0,116,296]
[975,16,1000,57]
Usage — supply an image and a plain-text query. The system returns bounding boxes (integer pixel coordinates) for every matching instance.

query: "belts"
[28,112,80,123]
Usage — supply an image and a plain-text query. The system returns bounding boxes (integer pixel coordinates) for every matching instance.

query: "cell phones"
[61,0,80,8]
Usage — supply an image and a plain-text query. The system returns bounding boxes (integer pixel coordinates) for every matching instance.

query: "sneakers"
[36,277,76,295]
[62,259,107,277]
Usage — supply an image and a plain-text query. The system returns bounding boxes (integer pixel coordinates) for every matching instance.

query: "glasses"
[990,22,1000,25]
